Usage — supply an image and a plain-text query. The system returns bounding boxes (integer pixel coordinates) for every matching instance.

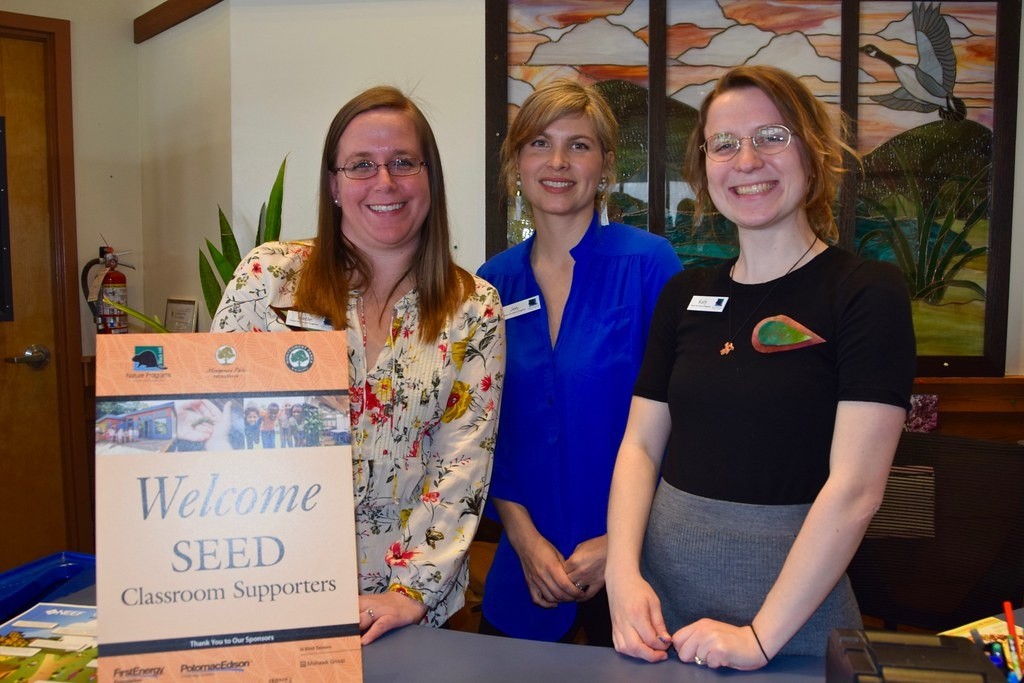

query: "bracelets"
[750,625,770,663]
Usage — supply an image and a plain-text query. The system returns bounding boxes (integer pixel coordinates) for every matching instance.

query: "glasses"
[332,155,428,180]
[699,123,798,163]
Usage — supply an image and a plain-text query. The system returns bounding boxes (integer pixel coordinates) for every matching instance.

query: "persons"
[475,78,684,643]
[244,400,321,448]
[605,66,917,669]
[100,402,244,448]
[210,86,505,646]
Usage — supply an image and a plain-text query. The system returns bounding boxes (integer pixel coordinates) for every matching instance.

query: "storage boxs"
[0,551,96,624]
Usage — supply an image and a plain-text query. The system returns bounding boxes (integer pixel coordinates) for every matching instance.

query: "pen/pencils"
[1007,635,1023,681]
[1003,601,1024,673]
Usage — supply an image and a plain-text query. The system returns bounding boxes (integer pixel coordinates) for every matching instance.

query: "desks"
[54,574,827,683]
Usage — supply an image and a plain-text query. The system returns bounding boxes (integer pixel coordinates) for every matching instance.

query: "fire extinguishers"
[80,246,136,334]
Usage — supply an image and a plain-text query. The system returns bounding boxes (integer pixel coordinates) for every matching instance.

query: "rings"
[575,582,581,589]
[366,609,374,621]
[694,657,702,664]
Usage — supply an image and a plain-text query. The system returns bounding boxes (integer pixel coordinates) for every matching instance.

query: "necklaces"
[721,237,817,355]
